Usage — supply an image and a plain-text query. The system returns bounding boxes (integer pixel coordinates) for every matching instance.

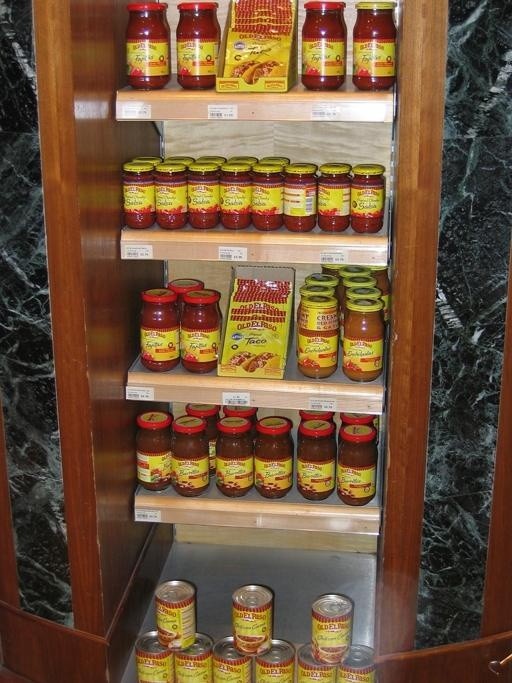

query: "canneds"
[337,425,378,505]
[284,163,318,232]
[342,273,377,296]
[251,164,284,230]
[180,289,222,373]
[307,273,338,288]
[172,415,209,496]
[260,157,289,167]
[123,162,155,229]
[174,633,212,683]
[352,2,397,90]
[256,639,295,683]
[164,156,193,167]
[215,416,254,497]
[339,285,382,347]
[176,3,222,89]
[220,163,251,230]
[312,593,354,665]
[342,295,385,382]
[155,163,187,230]
[213,637,253,683]
[254,416,294,498]
[135,411,172,491]
[337,645,375,683]
[319,264,344,277]
[136,631,173,683]
[338,413,380,447]
[297,420,337,501]
[168,279,203,316]
[186,403,221,477]
[134,156,162,165]
[297,642,336,682]
[372,266,391,325]
[124,4,170,88]
[227,156,257,165]
[232,584,273,657]
[297,283,336,322]
[155,580,196,652]
[298,296,339,380]
[187,163,220,229]
[351,164,386,233]
[140,289,180,371]
[195,156,226,166]
[300,3,347,90]
[341,264,371,278]
[222,405,258,441]
[318,163,350,232]
[298,411,336,437]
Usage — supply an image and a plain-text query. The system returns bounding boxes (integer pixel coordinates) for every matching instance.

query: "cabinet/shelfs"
[26,1,451,683]
